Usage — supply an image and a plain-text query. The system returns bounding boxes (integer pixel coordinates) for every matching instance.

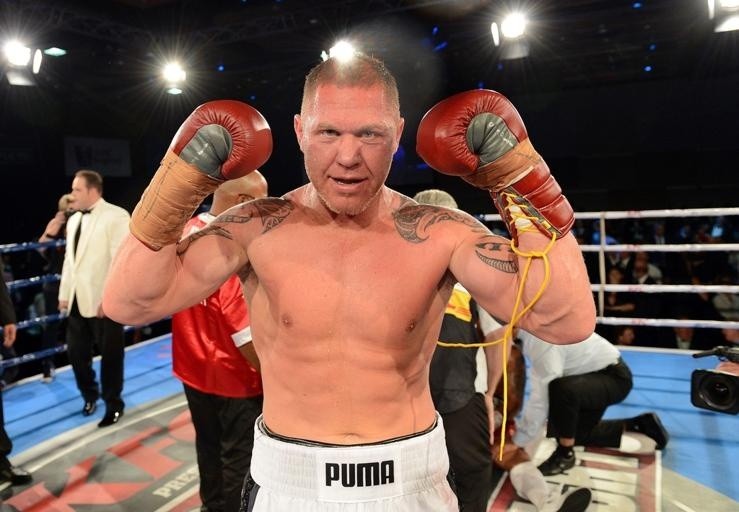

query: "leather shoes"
[538,445,577,474]
[97,407,123,427]
[83,400,95,416]
[1,463,31,484]
[637,411,670,451]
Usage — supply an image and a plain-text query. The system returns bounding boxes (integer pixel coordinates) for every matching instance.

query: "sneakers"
[540,486,591,511]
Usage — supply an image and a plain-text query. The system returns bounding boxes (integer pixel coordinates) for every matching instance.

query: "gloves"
[415,88,576,246]
[127,98,276,253]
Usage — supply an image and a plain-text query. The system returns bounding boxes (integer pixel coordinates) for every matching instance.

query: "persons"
[1,272,33,498]
[492,345,593,512]
[410,188,508,512]
[57,170,131,428]
[103,52,596,512]
[491,324,666,477]
[716,361,739,378]
[574,214,739,351]
[173,167,269,512]
[2,236,174,387]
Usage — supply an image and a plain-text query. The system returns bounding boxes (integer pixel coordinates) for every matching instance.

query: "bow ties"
[82,211,91,214]
